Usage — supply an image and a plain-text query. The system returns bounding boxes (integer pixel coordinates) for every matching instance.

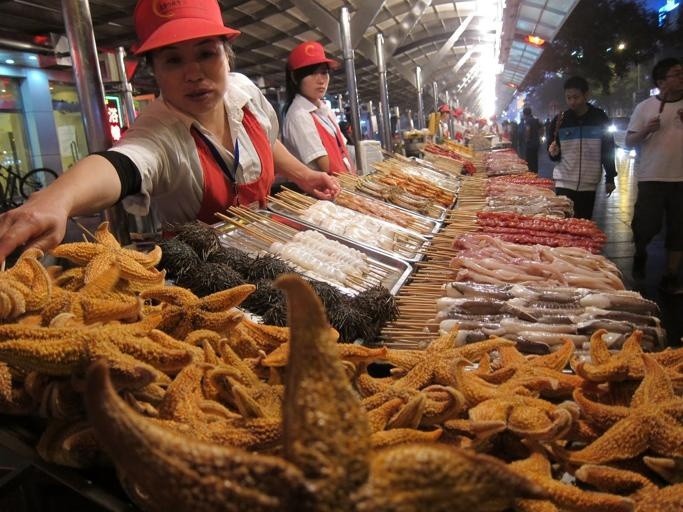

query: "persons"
[435,104,553,176]
[279,41,355,178]
[0,0,343,272]
[622,56,682,293]
[546,77,618,221]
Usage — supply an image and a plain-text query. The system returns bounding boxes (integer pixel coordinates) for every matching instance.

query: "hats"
[288,41,338,70]
[132,0,241,56]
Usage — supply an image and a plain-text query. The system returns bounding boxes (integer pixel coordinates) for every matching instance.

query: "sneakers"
[659,274,683,295]
[631,251,647,280]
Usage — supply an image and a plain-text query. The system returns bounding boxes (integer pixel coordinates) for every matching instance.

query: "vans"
[609,116,630,142]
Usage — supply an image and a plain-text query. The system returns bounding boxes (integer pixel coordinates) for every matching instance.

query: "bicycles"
[0,164,58,211]
